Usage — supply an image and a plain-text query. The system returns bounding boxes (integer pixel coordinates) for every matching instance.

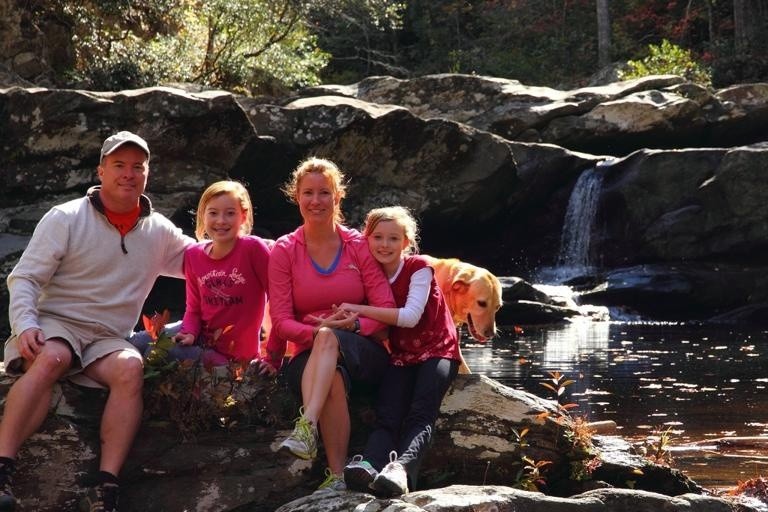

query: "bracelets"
[352,320,361,335]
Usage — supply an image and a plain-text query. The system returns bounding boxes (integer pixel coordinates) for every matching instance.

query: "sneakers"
[0,464,18,511]
[373,460,408,498]
[278,417,319,460]
[79,482,120,512]
[343,460,376,493]
[312,474,346,497]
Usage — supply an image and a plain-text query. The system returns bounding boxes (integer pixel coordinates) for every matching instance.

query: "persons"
[336,205,462,498]
[267,157,397,495]
[0,131,275,512]
[126,181,288,379]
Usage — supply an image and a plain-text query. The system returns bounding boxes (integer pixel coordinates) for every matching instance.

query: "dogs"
[416,252,506,375]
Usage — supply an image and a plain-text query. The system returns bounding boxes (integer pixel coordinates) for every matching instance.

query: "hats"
[99,130,151,164]
